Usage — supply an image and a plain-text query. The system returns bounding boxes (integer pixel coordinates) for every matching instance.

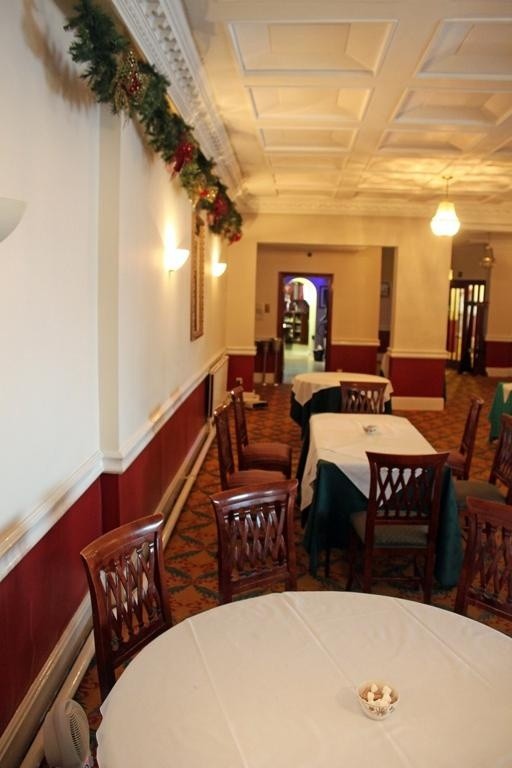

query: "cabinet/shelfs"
[282,312,308,344]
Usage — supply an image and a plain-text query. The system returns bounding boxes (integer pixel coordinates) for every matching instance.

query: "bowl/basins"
[357,680,400,720]
[363,425,377,435]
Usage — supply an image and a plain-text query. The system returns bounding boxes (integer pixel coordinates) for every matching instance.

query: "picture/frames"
[380,280,389,297]
[190,212,204,340]
[319,285,329,308]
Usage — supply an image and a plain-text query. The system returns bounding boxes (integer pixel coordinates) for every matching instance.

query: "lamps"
[430,175,460,236]
[212,232,227,278]
